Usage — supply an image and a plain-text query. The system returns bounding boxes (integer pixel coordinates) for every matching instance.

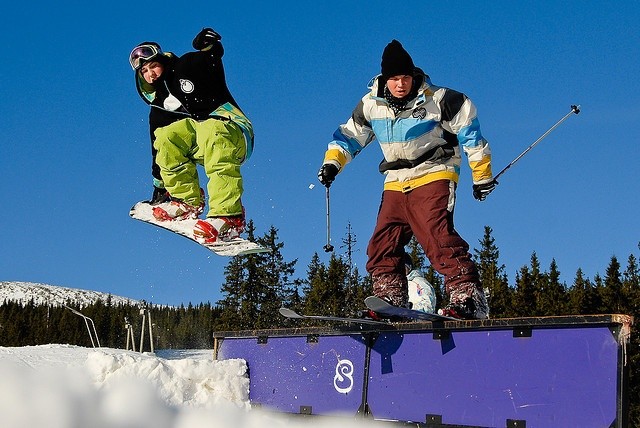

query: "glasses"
[129,45,163,70]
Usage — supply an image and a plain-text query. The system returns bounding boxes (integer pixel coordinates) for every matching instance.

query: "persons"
[403,251,436,315]
[130,27,256,244]
[320,40,495,318]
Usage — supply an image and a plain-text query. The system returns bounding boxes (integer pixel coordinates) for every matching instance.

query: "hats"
[381,39,414,83]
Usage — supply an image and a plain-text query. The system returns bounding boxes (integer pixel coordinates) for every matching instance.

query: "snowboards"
[129,198,274,259]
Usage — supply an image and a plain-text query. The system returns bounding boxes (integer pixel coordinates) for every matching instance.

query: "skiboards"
[279,296,463,325]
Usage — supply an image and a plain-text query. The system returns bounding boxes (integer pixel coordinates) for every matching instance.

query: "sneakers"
[194,206,246,238]
[370,289,412,310]
[154,188,206,221]
[439,298,489,320]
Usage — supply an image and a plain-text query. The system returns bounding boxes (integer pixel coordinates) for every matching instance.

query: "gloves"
[473,182,495,201]
[318,164,339,185]
[152,187,169,203]
[193,28,222,49]
